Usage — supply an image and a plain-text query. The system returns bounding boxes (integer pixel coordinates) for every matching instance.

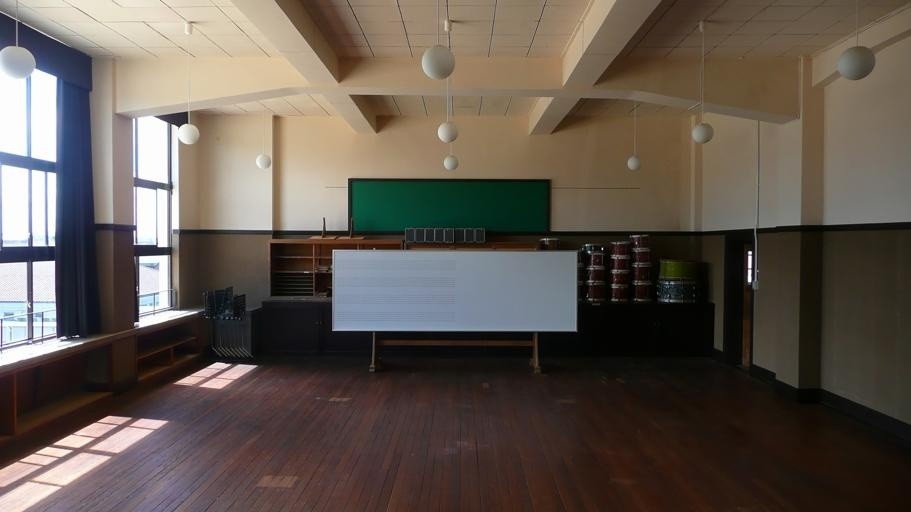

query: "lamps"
[255,108,272,171]
[627,98,641,171]
[839,3,876,82]
[178,21,199,145]
[0,0,34,84]
[422,0,459,171]
[691,18,714,144]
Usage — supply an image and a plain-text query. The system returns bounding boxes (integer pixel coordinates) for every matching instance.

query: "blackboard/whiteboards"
[347,178,551,235]
[331,249,579,334]
[405,227,486,243]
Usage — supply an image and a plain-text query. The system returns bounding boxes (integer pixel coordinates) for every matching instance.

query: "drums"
[655,279,701,304]
[629,234,650,250]
[657,257,704,280]
[577,240,655,304]
[538,237,565,252]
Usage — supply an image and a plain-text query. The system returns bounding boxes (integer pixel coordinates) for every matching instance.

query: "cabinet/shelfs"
[259,301,715,357]
[0,303,208,444]
[268,237,541,298]
